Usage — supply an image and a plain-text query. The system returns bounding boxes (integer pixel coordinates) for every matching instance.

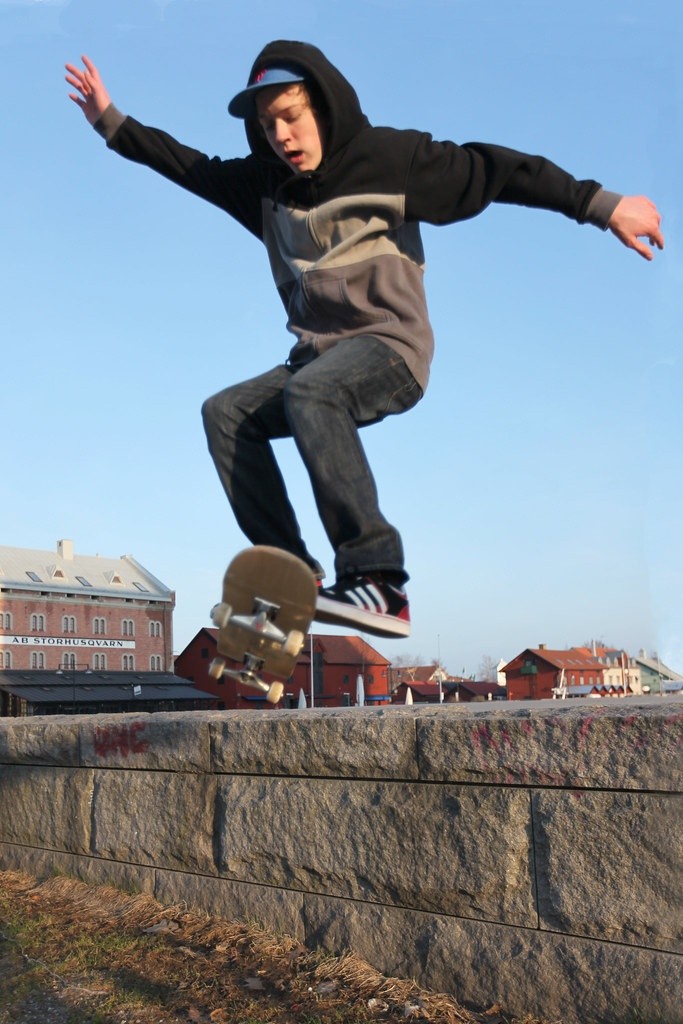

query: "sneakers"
[309,576,411,639]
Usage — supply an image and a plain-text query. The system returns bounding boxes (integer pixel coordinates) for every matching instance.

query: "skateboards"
[208,543,319,706]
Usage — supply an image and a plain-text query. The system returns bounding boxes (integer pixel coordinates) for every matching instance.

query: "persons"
[64,41,667,636]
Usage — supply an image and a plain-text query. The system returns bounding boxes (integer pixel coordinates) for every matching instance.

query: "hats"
[227,65,308,118]
